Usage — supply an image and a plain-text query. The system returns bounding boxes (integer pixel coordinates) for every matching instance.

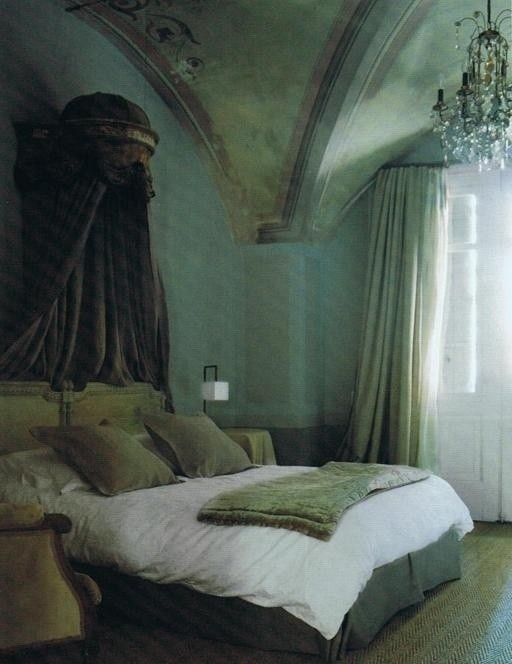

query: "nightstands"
[222,427,280,464]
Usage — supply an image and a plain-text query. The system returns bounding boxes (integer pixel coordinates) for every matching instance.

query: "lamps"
[196,363,233,423]
[429,0,510,171]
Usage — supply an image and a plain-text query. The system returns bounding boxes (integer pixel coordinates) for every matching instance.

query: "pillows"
[146,411,249,479]
[38,425,180,496]
[1,447,89,495]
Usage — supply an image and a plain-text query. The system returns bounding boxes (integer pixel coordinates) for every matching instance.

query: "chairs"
[0,514,105,663]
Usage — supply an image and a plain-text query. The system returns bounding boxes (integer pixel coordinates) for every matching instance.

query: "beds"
[0,378,475,663]
